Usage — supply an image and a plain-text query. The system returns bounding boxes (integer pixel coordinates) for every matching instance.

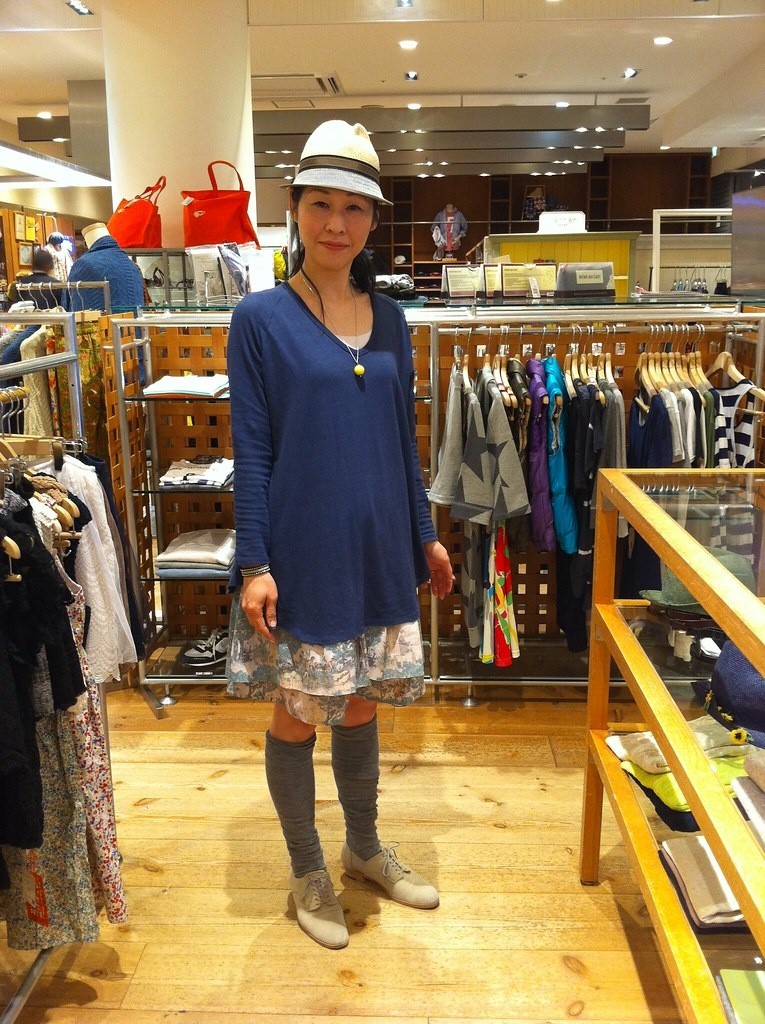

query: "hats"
[638,545,756,616]
[279,120,394,207]
[691,640,765,749]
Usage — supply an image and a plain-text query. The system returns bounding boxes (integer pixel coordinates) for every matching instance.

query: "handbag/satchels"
[106,176,166,248]
[181,161,261,251]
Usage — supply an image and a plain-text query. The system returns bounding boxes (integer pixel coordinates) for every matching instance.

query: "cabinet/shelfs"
[580,468,765,1023]
[111,315,765,691]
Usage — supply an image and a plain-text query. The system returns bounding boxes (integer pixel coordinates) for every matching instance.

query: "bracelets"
[240,563,270,577]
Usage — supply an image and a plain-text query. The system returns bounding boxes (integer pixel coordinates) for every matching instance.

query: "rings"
[431,570,435,574]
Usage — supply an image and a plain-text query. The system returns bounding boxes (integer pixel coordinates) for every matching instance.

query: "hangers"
[6,281,100,323]
[0,385,88,583]
[671,264,727,290]
[450,325,765,412]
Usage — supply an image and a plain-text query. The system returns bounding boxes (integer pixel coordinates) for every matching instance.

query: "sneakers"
[181,627,229,666]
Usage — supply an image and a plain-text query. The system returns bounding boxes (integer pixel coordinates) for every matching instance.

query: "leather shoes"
[289,867,349,949]
[341,840,440,909]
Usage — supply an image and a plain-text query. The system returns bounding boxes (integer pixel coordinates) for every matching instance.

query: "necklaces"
[299,269,365,378]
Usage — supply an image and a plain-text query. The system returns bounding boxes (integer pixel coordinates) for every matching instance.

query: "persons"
[5,249,71,313]
[62,220,146,391]
[42,231,75,284]
[225,118,458,957]
[431,204,468,253]
